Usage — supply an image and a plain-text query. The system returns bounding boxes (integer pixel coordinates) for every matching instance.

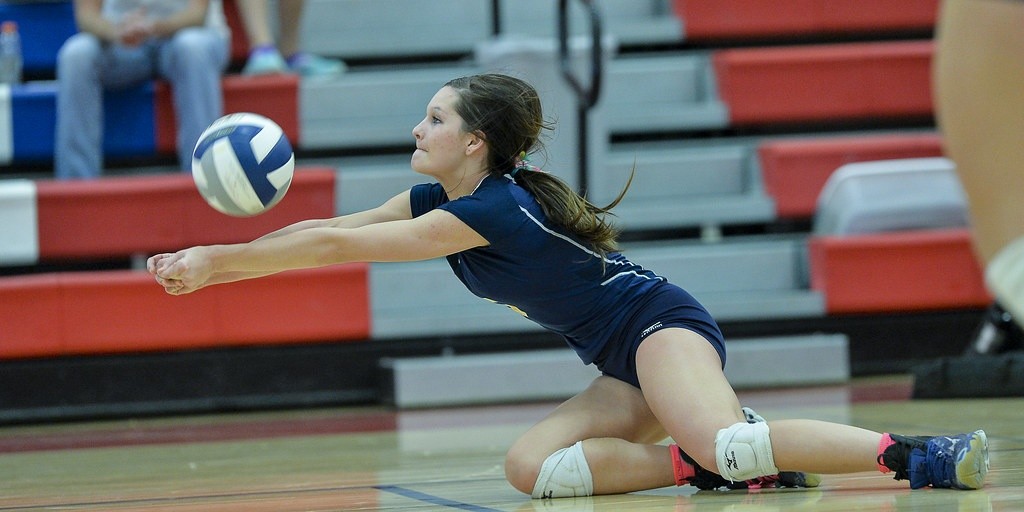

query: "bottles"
[0,20,22,84]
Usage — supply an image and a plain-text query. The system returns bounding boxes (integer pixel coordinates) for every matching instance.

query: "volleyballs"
[191,112,294,218]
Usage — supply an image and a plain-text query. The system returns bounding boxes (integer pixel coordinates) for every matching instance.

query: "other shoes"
[241,42,349,76]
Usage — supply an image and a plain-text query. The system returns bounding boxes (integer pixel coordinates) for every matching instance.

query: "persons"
[146,74,990,498]
[56,0,232,180]
[931,0,1024,331]
[237,0,349,75]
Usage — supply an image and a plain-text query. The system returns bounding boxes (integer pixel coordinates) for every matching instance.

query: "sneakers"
[909,429,989,490]
[678,446,822,491]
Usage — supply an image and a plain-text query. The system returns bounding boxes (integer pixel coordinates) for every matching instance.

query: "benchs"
[0,0,998,427]
